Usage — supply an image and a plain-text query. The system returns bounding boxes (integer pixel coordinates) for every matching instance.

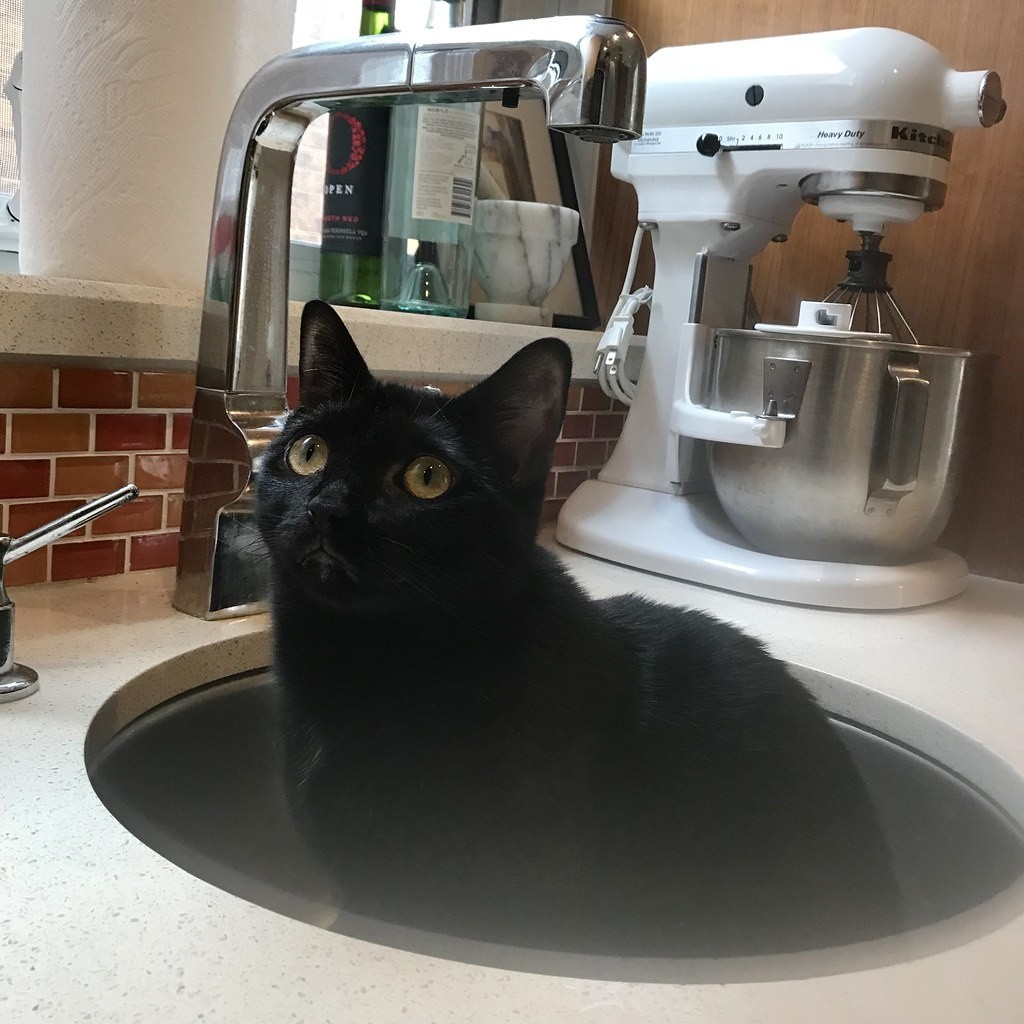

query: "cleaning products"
[380,102,481,318]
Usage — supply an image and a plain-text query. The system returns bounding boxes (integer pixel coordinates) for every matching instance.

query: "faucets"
[168,14,648,620]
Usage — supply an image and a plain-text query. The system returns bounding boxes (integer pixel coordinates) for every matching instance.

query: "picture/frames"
[466,97,604,330]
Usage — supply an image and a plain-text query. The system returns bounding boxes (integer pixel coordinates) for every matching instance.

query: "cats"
[249,296,909,957]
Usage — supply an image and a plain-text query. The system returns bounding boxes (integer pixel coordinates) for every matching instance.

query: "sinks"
[81,624,1024,982]
